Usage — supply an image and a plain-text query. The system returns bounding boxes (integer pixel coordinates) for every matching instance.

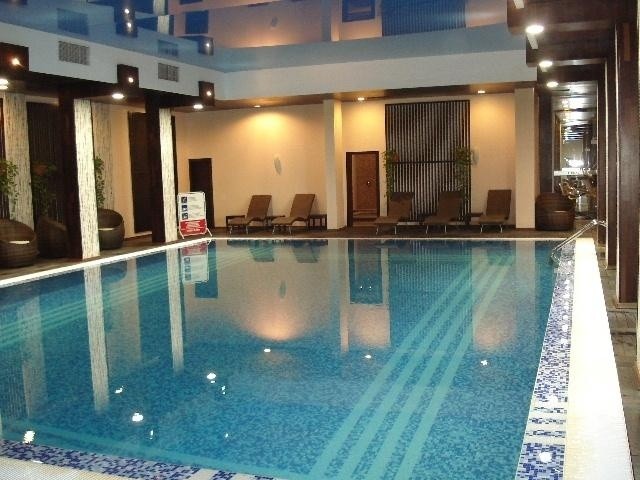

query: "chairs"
[227,192,315,236]
[0,208,124,269]
[535,191,574,231]
[372,189,512,235]
